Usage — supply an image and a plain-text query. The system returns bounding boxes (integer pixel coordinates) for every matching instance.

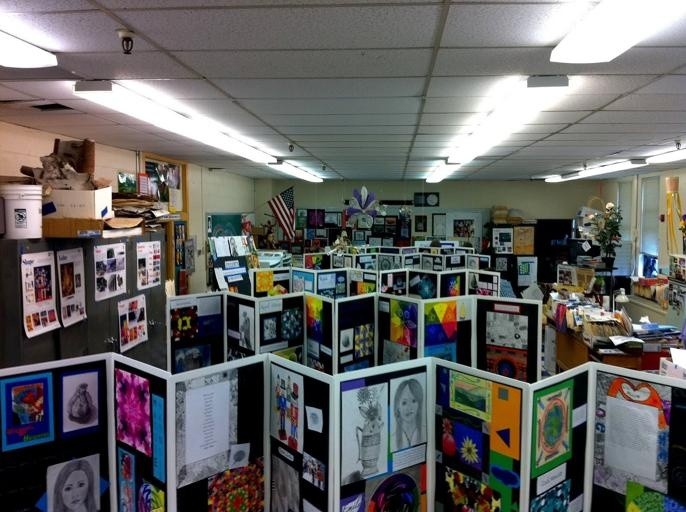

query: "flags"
[267,186,295,243]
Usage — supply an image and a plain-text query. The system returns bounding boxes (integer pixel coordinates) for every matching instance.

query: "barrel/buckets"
[2,185,42,239]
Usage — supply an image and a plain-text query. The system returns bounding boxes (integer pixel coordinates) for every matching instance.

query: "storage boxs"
[40,215,102,239]
[38,179,115,221]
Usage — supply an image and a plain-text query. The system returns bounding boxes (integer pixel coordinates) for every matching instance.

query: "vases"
[602,256,616,268]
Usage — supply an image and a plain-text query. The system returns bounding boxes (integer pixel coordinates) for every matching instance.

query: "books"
[546,287,682,355]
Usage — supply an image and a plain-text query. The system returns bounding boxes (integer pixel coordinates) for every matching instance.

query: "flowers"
[579,201,626,257]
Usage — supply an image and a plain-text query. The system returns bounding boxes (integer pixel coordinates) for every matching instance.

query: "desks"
[546,315,647,374]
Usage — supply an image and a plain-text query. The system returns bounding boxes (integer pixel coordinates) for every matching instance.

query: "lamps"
[71,80,323,183]
[545,136,686,184]
[612,287,630,317]
[550,0,686,64]
[426,76,583,184]
[0,30,58,68]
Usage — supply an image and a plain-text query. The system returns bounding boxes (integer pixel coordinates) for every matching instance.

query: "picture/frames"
[136,149,189,223]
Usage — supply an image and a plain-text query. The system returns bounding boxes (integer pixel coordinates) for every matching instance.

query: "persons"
[54,459,98,512]
[390,378,426,452]
[260,219,278,241]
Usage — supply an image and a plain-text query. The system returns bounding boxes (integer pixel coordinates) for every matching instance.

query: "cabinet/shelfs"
[0,228,167,373]
